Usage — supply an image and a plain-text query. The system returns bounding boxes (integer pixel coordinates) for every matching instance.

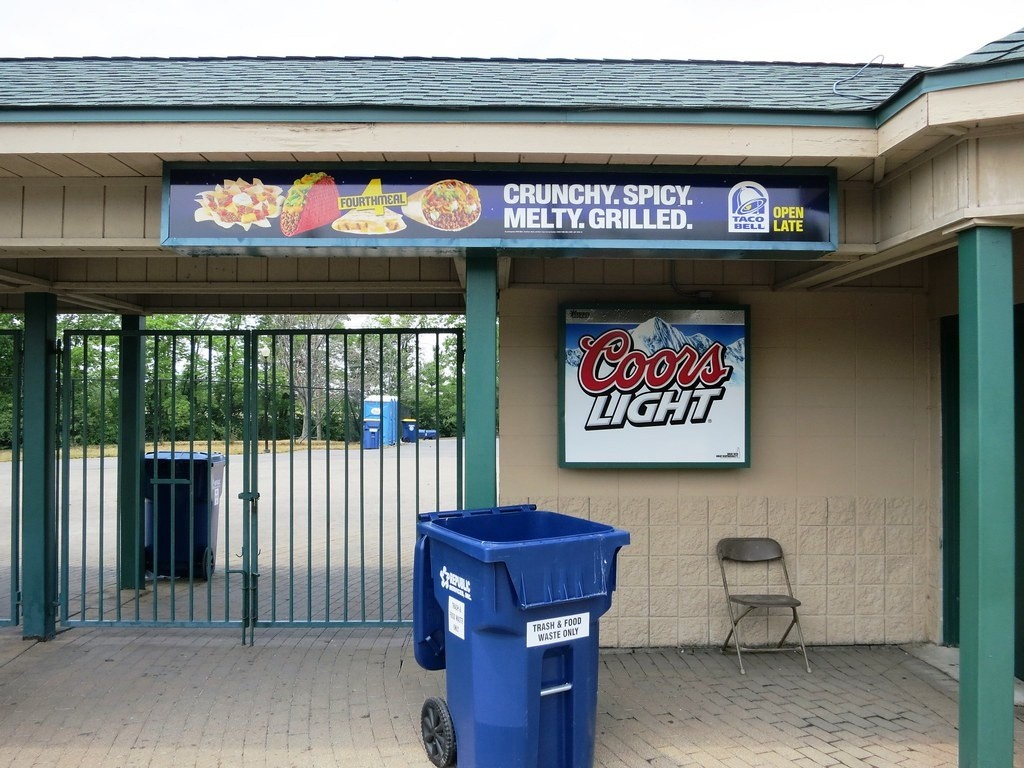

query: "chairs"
[717,538,813,675]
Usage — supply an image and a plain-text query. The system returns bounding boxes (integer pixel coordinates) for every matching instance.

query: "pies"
[401,179,482,232]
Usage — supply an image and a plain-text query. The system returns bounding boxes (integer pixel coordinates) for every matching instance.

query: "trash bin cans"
[145,449,226,582]
[400,419,417,442]
[363,419,380,449]
[411,503,632,768]
